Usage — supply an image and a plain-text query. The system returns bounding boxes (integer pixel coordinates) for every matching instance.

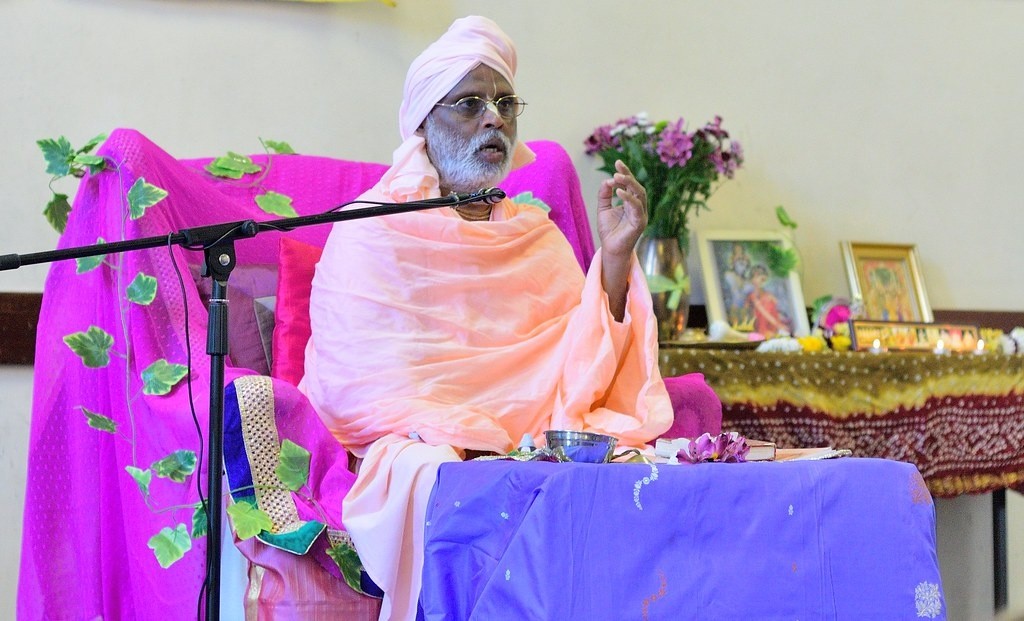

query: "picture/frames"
[841,240,934,323]
[698,229,810,341]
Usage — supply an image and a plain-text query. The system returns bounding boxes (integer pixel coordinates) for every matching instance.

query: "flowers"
[586,112,744,240]
[677,430,751,463]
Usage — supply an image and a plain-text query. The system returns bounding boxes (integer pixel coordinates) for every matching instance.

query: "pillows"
[271,236,322,389]
[188,262,279,379]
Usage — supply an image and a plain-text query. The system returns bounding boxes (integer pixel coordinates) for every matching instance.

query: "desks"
[655,348,1024,615]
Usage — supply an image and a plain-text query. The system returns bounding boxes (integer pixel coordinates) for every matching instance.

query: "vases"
[637,237,689,341]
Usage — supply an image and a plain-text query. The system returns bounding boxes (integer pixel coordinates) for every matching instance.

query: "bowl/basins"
[542,429,620,464]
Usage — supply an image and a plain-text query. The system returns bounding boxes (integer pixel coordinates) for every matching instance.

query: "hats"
[398,16,515,138]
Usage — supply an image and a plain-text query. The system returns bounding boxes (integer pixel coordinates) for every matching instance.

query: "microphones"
[478,186,506,204]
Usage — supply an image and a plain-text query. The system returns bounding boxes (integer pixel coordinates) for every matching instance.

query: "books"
[655,436,776,463]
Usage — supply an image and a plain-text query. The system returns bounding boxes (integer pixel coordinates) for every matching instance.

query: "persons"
[298,15,674,621]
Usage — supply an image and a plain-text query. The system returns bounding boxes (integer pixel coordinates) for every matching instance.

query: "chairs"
[109,128,721,620]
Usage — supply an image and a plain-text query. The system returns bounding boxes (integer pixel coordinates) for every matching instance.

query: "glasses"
[434,95,528,118]
